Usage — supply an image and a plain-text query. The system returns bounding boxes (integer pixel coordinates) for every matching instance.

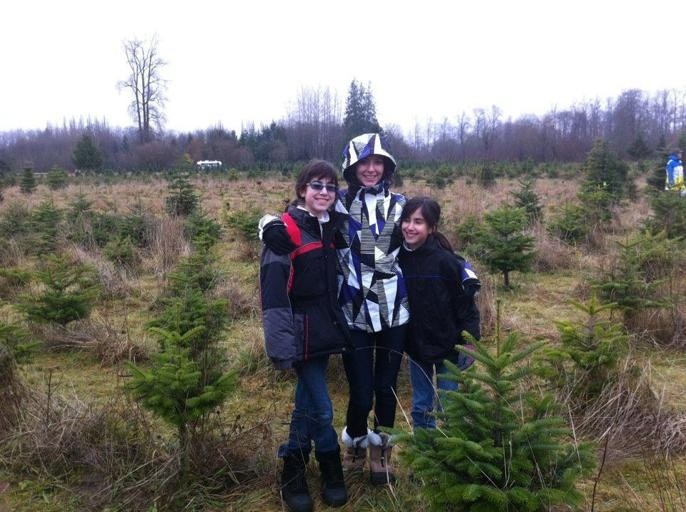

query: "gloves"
[261,219,296,256]
[456,345,477,370]
[457,283,477,302]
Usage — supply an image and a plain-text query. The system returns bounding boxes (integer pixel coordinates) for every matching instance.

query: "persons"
[395,197,481,483]
[256,159,348,512]
[664,148,685,197]
[258,131,481,485]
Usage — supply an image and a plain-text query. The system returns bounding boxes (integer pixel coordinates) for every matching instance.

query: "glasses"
[305,180,339,193]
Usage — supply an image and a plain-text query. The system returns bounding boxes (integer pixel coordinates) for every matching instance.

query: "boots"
[341,425,369,482]
[278,444,315,512]
[315,444,348,507]
[366,428,397,487]
